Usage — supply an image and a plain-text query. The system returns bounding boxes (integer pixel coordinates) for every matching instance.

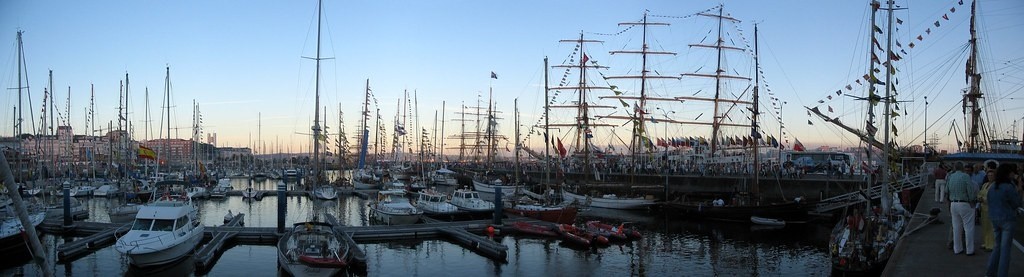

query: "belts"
[950,200,967,202]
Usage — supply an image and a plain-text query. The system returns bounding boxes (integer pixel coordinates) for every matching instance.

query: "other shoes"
[979,246,986,249]
[975,219,979,223]
[967,252,975,256]
[954,250,964,255]
[947,243,952,248]
[984,249,992,252]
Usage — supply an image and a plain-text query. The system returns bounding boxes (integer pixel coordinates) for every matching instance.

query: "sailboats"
[251,0,1024,271]
[0,30,231,266]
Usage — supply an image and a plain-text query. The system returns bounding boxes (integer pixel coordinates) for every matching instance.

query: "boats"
[276,211,367,277]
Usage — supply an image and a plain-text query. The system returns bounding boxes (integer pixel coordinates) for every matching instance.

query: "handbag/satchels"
[976,203,981,217]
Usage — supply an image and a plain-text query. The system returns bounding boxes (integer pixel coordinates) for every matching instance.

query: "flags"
[491,72,497,78]
[506,147,510,152]
[398,126,406,135]
[198,162,206,180]
[490,115,497,127]
[138,145,157,160]
[517,1,963,177]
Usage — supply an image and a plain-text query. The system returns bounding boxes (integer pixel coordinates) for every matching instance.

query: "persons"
[509,160,1024,277]
